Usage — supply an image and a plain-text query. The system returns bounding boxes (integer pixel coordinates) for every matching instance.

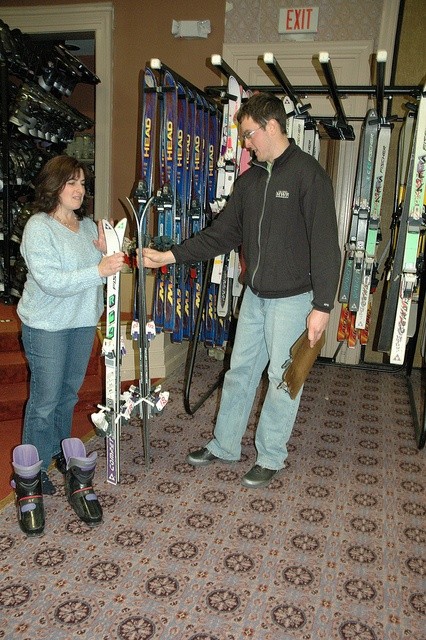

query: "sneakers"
[185,445,240,466]
[241,465,280,489]
[53,454,64,474]
[39,470,56,497]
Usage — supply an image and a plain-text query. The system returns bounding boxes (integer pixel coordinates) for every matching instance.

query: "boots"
[9,445,45,536]
[62,437,104,527]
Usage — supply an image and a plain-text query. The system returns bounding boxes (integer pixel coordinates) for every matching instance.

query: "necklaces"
[52,211,77,228]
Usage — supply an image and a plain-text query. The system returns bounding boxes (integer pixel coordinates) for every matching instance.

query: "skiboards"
[157,68,321,366]
[337,80,426,367]
[130,65,156,340]
[90,217,135,486]
[125,197,169,473]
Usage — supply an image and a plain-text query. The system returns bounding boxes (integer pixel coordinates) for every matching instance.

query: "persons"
[16,155,126,494]
[136,93,342,486]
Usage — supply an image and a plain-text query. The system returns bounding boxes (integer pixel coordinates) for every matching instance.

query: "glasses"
[237,122,267,144]
